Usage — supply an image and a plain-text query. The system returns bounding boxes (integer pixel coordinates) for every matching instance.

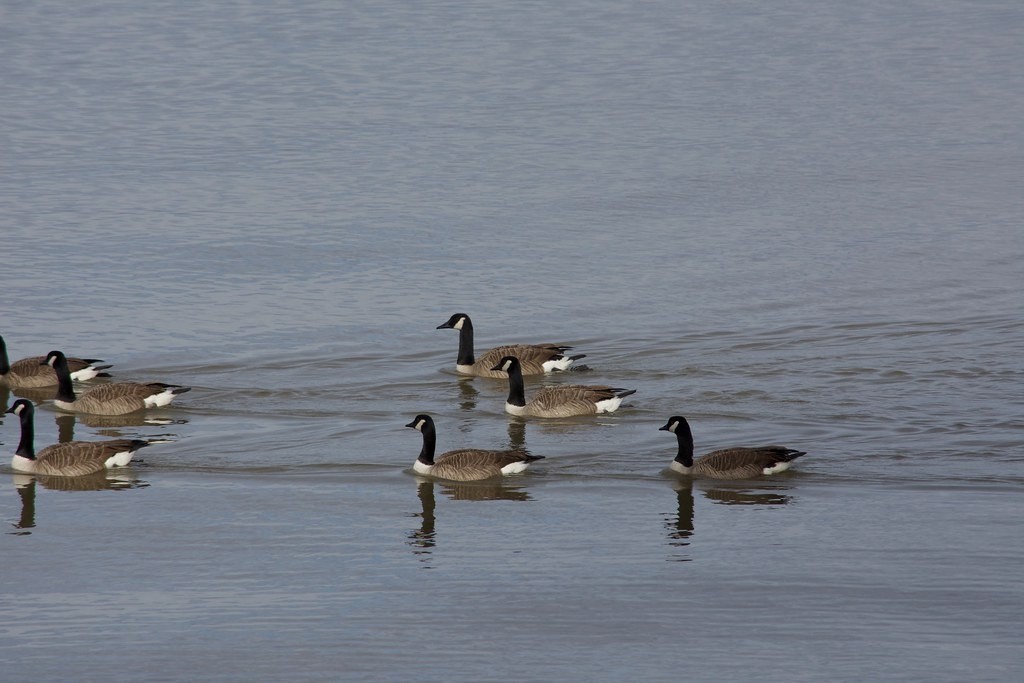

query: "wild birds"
[658,416,807,475]
[489,356,637,417]
[0,335,114,389]
[405,414,546,481]
[436,312,587,378]
[39,351,191,416]
[4,398,150,477]
